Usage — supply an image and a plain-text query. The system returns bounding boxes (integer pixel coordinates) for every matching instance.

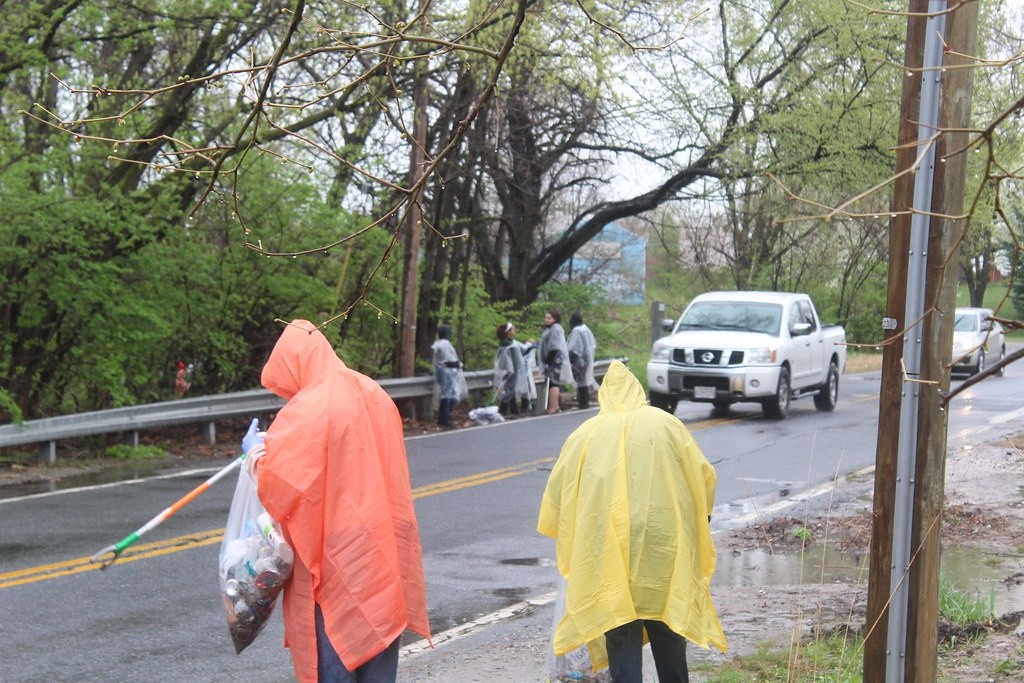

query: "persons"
[524,338,540,402]
[241,319,427,683]
[495,321,535,419]
[434,324,465,428]
[541,360,716,683]
[535,309,566,416]
[565,310,596,411]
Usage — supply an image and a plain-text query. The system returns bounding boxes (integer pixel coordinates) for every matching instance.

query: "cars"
[950,307,1009,377]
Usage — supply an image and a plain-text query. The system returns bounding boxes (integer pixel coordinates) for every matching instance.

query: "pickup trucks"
[646,291,848,420]
